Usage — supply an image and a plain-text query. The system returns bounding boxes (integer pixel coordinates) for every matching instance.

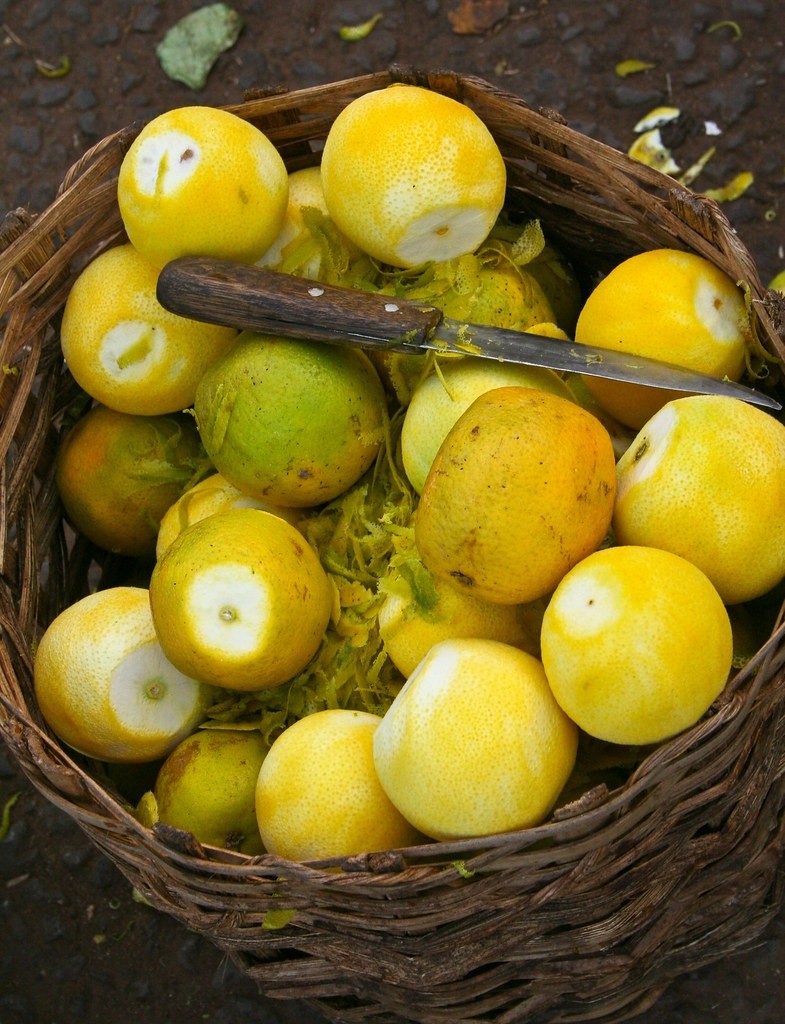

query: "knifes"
[156,254,782,411]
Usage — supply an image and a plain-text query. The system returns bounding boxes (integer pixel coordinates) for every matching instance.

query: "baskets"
[2,68,785,1024]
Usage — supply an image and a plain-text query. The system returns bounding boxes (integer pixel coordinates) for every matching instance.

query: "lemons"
[28,79,784,870]
